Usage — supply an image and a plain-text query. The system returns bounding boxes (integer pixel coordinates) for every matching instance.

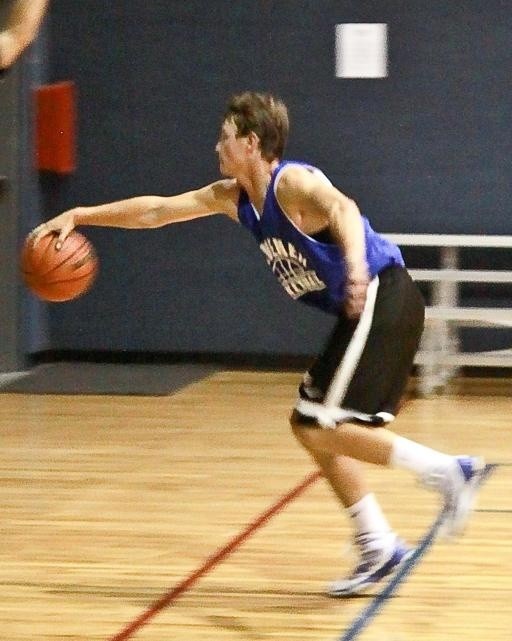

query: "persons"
[27,89,488,602]
[0,0,52,73]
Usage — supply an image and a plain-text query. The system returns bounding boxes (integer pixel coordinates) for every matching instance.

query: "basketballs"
[20,230,98,302]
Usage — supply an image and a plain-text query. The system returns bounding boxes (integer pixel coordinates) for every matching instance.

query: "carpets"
[0,358,225,397]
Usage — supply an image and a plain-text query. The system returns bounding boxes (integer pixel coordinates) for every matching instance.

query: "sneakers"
[328,531,410,598]
[428,454,482,536]
